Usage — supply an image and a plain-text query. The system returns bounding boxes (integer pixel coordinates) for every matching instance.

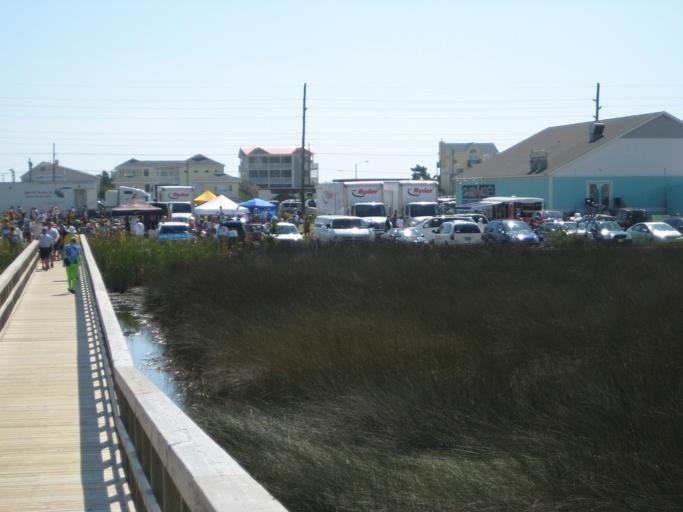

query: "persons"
[80,205,167,237]
[384,210,398,232]
[186,206,312,236]
[1,206,76,269]
[63,238,82,293]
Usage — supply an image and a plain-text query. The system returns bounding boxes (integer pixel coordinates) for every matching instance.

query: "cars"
[281,198,316,216]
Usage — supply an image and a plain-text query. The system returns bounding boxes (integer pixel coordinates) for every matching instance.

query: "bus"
[484,196,544,223]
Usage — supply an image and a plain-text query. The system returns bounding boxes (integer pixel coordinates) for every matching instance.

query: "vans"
[438,198,456,214]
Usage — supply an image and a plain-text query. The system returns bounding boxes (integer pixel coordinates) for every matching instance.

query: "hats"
[67,226,77,233]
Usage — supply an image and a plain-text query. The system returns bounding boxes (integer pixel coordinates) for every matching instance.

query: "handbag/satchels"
[64,255,71,266]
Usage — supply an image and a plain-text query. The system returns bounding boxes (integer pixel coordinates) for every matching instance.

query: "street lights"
[355,161,368,178]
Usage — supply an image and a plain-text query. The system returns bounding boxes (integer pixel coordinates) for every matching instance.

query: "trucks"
[150,184,197,230]
[315,180,440,232]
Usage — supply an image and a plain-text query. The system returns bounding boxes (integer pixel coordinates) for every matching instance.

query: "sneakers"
[42,263,53,271]
[67,288,76,294]
